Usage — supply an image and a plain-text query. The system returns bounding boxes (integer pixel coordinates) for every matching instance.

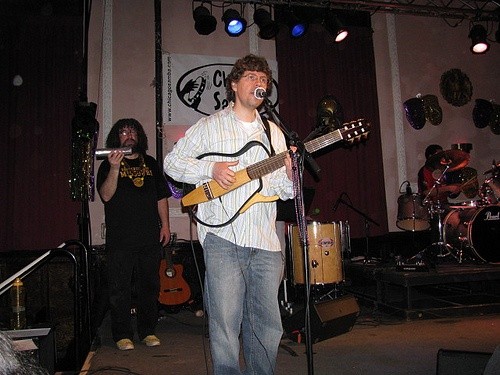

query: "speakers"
[282,293,361,344]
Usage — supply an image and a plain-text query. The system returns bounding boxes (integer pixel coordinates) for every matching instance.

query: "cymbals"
[425,149,469,170]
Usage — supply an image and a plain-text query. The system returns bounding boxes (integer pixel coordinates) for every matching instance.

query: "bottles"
[11,278,25,330]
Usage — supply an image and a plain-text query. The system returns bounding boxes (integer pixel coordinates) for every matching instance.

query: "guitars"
[180,117,374,228]
[157,232,192,305]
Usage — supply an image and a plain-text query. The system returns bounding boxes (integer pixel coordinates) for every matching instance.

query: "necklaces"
[120,155,148,187]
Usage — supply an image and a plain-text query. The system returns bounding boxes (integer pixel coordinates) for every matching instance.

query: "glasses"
[119,129,137,136]
[240,73,269,84]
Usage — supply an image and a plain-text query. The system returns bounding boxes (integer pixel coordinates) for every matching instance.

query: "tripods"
[420,169,463,264]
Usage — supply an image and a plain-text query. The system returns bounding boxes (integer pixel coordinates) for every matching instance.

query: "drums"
[396,193,431,233]
[478,172,500,204]
[442,166,479,189]
[443,204,500,265]
[288,221,350,286]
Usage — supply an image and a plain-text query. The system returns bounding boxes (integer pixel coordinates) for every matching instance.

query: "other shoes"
[140,334,161,347]
[116,338,134,351]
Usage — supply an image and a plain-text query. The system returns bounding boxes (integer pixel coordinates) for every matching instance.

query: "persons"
[163,54,302,375]
[418,144,475,237]
[97,118,170,350]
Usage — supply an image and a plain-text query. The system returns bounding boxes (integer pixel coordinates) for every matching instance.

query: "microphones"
[253,86,269,99]
[332,192,343,213]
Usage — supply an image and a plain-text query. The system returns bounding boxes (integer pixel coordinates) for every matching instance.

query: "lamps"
[468,16,491,55]
[192,0,351,44]
